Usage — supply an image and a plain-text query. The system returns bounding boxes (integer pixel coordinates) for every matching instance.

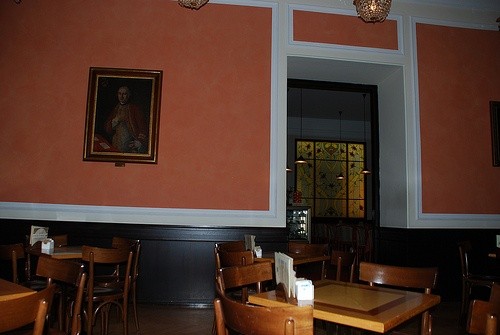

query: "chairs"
[37,256,86,334]
[358,262,438,293]
[320,222,372,281]
[215,239,254,265]
[468,285,499,335]
[0,283,55,335]
[212,265,313,335]
[81,245,130,335]
[101,237,141,333]
[288,243,326,256]
[462,247,494,298]
[25,236,67,247]
[2,244,46,289]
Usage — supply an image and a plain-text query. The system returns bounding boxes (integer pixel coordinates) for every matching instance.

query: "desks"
[47,245,81,259]
[249,280,440,333]
[253,253,330,265]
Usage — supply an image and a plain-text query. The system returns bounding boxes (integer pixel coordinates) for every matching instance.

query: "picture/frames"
[82,66,163,163]
[489,101,499,168]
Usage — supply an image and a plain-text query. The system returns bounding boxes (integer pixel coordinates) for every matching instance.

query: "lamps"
[179,0,209,10]
[337,110,345,181]
[353,0,392,23]
[360,93,372,174]
[294,86,310,164]
[286,87,292,172]
